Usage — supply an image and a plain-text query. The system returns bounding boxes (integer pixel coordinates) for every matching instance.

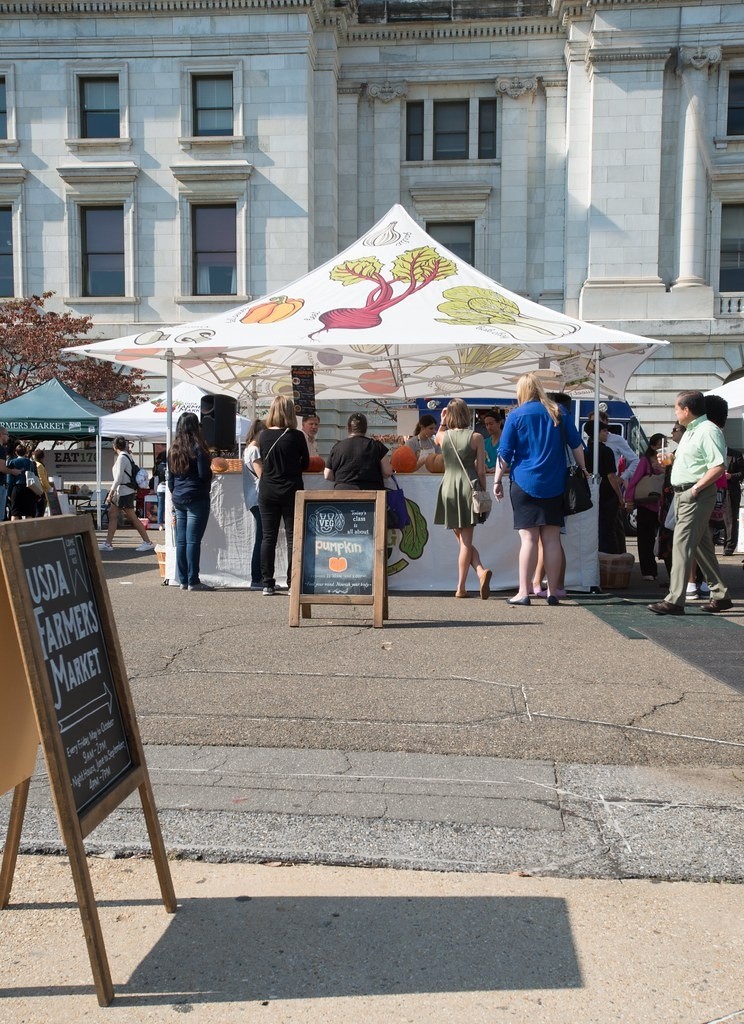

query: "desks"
[169,473,601,595]
[68,495,91,511]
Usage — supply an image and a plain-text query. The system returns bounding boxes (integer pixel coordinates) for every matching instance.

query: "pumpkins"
[425,452,445,473]
[305,456,325,472]
[211,457,230,472]
[391,445,417,473]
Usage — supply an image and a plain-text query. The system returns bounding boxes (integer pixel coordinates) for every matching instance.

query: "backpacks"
[122,453,141,490]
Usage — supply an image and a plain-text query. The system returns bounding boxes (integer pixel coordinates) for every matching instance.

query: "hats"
[585,420,611,435]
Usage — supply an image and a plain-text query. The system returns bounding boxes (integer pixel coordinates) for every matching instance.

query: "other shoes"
[480,570,493,600]
[547,596,559,605]
[456,591,470,598]
[529,586,543,594]
[263,586,276,595]
[685,589,699,599]
[188,582,215,591]
[643,576,657,580]
[723,550,732,556]
[538,588,566,597]
[251,582,280,591]
[180,583,189,590]
[700,588,710,598]
[506,596,530,605]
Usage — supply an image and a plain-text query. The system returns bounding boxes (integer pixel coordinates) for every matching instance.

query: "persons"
[167,411,217,592]
[98,436,155,552]
[481,412,513,473]
[240,419,282,589]
[131,448,166,531]
[323,410,393,490]
[403,414,443,473]
[433,398,493,600]
[0,425,53,522]
[257,395,310,595]
[300,414,320,456]
[580,390,744,615]
[493,372,590,606]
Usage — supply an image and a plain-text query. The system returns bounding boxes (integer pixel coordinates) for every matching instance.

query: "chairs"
[78,489,111,528]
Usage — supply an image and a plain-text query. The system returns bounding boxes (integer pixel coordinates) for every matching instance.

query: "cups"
[656,447,671,465]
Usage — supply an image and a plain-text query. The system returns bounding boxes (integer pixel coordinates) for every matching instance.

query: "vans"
[412,393,659,538]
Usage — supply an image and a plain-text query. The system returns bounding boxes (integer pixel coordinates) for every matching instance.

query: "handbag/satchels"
[559,415,594,517]
[385,475,410,530]
[25,461,43,495]
[471,490,492,514]
[633,456,666,502]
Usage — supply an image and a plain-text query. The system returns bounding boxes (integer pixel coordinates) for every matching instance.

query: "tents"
[59,201,672,470]
[0,377,116,529]
[98,381,250,531]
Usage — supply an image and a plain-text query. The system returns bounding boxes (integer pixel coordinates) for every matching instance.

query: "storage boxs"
[139,518,148,529]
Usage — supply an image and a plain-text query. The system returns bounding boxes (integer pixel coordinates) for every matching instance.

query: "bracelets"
[493,481,502,484]
[692,485,701,492]
[108,494,113,498]
[582,468,586,471]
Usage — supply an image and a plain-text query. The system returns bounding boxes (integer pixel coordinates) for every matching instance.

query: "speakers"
[545,392,573,415]
[198,394,236,451]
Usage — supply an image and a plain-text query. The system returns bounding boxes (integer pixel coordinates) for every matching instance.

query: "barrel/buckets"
[599,555,635,590]
[156,551,166,578]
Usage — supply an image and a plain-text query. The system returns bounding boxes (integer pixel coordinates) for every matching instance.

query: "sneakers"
[99,542,113,552]
[135,542,154,551]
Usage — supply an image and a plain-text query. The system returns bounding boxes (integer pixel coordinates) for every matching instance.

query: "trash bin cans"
[156,552,166,578]
[599,556,633,589]
[139,519,149,529]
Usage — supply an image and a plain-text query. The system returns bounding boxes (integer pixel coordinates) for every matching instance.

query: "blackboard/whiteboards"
[47,492,62,515]
[0,513,149,838]
[291,490,388,604]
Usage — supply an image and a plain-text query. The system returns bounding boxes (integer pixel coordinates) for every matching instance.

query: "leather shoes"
[700,598,732,612]
[647,600,685,615]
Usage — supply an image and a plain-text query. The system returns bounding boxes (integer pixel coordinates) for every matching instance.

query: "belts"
[672,483,694,492]
